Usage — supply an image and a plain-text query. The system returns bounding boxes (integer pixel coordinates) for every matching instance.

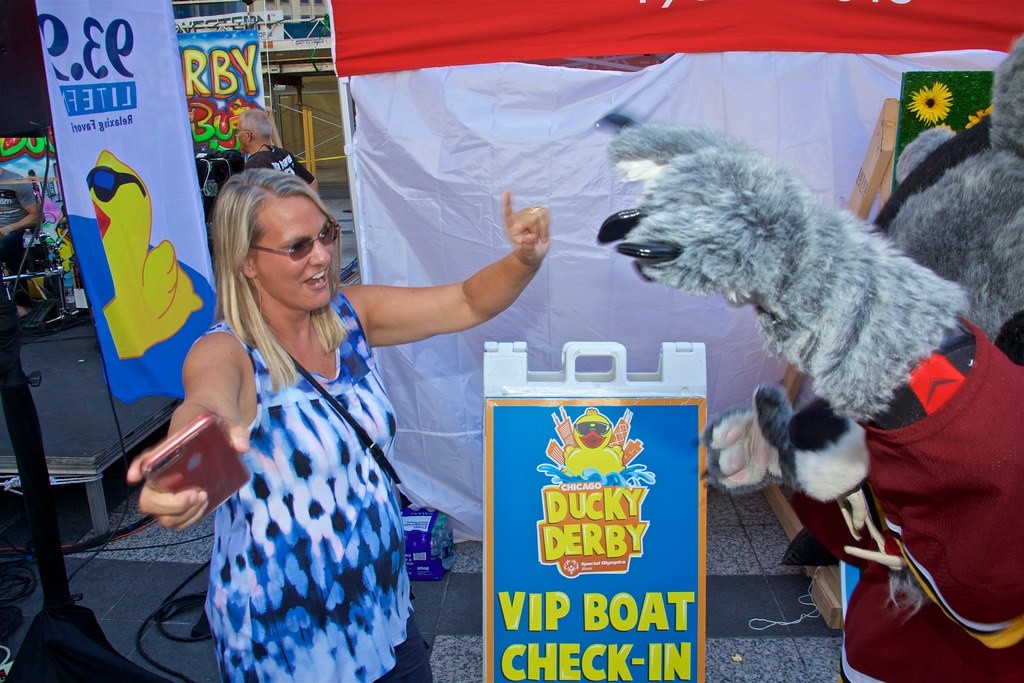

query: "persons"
[125,163,551,682]
[235,109,317,188]
[0,166,40,320]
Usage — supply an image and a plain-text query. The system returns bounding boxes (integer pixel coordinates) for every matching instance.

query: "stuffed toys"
[596,33,1024,683]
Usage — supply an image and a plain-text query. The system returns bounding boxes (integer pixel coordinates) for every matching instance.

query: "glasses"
[234,128,249,136]
[247,219,343,260]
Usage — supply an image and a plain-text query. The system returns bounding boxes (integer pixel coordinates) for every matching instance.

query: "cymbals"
[0,175,62,187]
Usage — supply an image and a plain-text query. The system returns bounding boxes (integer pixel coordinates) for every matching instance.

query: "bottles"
[424,510,456,580]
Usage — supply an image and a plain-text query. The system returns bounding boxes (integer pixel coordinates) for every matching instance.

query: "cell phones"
[139,412,251,523]
